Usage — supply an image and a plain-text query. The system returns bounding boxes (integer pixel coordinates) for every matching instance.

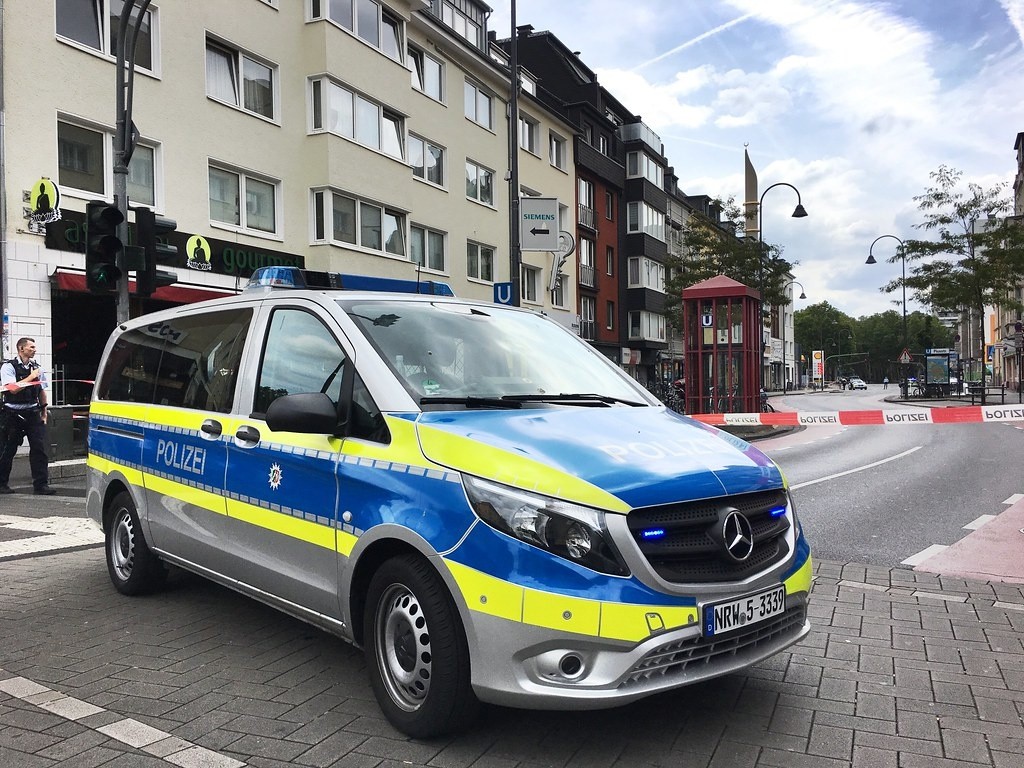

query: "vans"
[86,268,812,743]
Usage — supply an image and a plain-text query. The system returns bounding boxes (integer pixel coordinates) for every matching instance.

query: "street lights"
[783,281,807,394]
[825,338,837,377]
[836,329,853,383]
[865,235,908,399]
[821,313,838,391]
[759,183,808,389]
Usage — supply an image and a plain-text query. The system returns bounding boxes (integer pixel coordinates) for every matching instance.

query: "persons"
[884,377,888,389]
[0,337,56,496]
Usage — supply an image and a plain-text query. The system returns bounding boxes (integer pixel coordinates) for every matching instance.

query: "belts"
[1,403,48,413]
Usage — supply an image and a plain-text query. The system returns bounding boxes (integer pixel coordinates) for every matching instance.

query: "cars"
[849,379,867,390]
[950,377,958,390]
[0,379,96,443]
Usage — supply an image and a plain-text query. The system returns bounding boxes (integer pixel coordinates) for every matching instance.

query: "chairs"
[404,339,466,391]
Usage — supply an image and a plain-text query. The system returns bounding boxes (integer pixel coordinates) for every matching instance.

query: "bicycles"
[965,382,990,397]
[759,385,776,413]
[639,378,686,415]
[913,382,930,398]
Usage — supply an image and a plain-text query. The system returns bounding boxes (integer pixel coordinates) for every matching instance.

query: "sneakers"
[33,480,56,495]
[0,485,15,494]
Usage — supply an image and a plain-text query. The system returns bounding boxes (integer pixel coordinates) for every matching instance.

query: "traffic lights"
[135,207,177,297]
[86,200,122,290]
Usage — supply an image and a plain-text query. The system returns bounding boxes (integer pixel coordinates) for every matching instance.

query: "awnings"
[50,273,233,304]
